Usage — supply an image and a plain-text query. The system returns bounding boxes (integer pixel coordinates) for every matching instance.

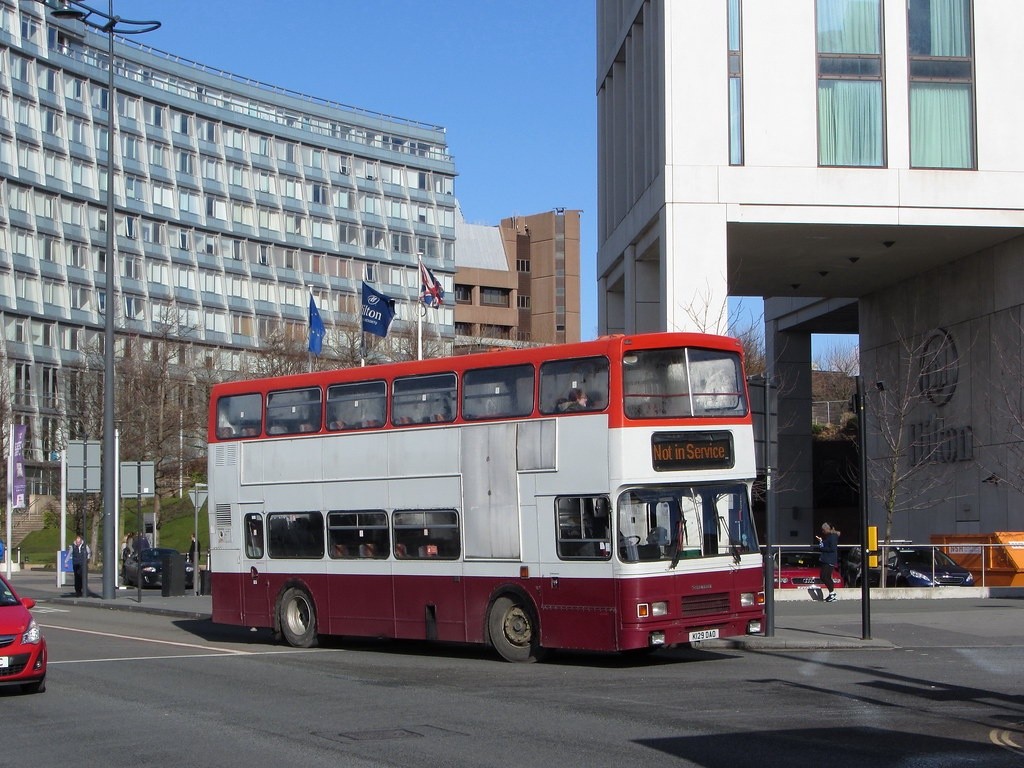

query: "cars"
[0,573,48,693]
[125,547,194,589]
[771,550,846,592]
[842,540,974,588]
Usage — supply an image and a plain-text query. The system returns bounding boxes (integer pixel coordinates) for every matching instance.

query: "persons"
[189,534,202,562]
[594,518,630,560]
[126,530,150,558]
[558,389,589,412]
[65,537,91,596]
[817,522,840,602]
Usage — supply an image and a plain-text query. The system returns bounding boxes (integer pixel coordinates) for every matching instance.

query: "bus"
[206,332,766,664]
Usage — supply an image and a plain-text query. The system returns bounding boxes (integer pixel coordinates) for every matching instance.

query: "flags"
[418,259,445,309]
[308,294,326,355]
[362,281,396,338]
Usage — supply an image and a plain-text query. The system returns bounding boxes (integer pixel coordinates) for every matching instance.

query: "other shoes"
[827,592,837,602]
